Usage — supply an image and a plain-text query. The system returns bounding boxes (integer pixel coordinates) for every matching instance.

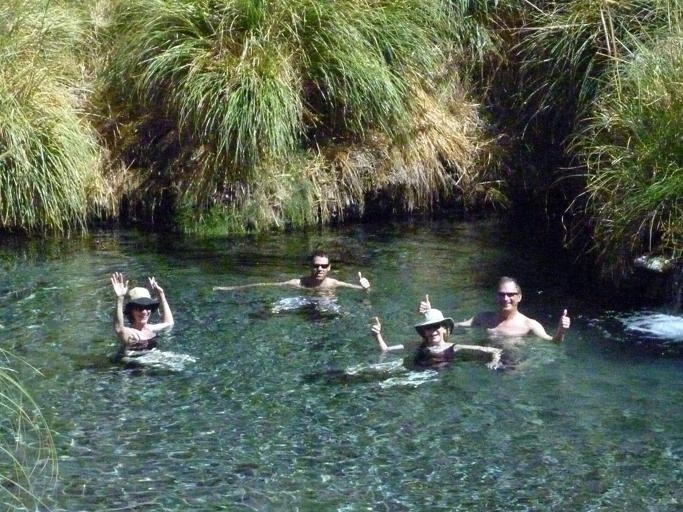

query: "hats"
[414,309,455,338]
[125,286,160,315]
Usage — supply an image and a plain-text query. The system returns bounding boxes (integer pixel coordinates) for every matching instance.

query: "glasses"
[499,292,518,297]
[313,264,329,268]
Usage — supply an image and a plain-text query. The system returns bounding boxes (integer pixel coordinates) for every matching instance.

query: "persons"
[110,271,174,377]
[213,251,370,317]
[342,276,571,389]
